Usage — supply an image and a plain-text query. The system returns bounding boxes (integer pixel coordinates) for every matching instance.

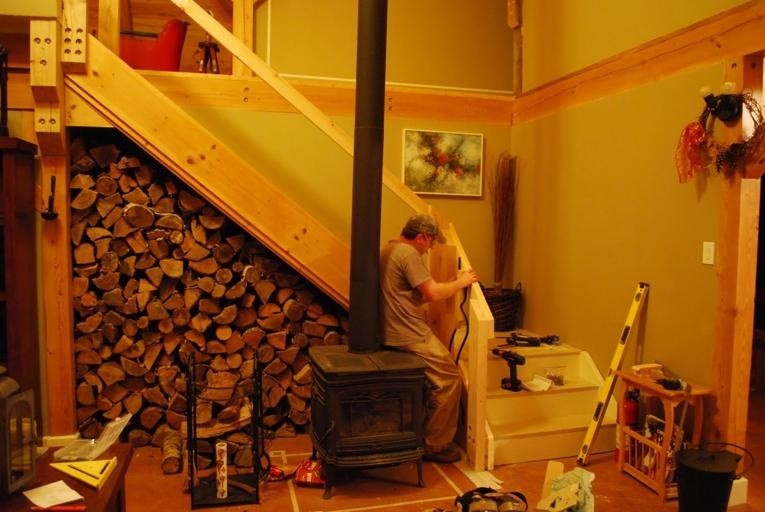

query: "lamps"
[700,82,738,121]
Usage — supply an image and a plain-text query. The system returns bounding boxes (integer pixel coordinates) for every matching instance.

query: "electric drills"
[488,348,525,391]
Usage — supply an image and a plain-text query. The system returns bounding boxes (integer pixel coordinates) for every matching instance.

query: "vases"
[480,282,521,331]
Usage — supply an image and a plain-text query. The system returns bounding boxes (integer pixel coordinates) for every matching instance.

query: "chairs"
[122,19,186,71]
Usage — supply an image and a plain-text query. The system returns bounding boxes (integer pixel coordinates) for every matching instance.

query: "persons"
[379,214,481,465]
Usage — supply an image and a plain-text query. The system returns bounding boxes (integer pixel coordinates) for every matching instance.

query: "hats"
[405,213,448,244]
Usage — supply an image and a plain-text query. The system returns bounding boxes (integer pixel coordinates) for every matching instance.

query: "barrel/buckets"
[674,442,753,512]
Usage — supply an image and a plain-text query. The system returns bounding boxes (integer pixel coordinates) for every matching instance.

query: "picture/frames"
[402,128,484,197]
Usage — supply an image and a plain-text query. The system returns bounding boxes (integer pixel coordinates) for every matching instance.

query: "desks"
[0,443,135,512]
[612,369,714,503]
